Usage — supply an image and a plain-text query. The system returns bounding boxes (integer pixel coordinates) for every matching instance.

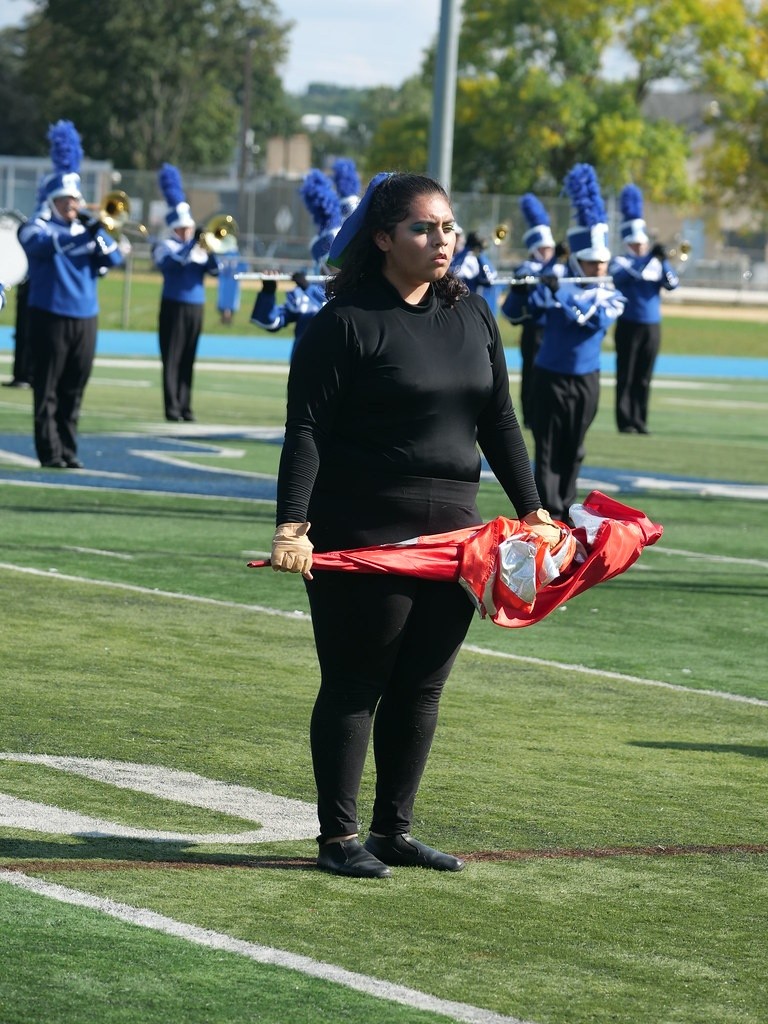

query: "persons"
[2,274,33,389]
[250,193,686,533]
[268,171,560,882]
[148,201,230,423]
[19,174,132,470]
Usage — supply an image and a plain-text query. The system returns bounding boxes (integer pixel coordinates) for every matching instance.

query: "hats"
[618,183,650,258]
[157,162,196,243]
[331,158,363,224]
[298,168,345,275]
[517,192,555,260]
[34,119,87,222]
[559,162,611,287]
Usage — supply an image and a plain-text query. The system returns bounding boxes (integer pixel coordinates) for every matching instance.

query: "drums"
[0,206,33,290]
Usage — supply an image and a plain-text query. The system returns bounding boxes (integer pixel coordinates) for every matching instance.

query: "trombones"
[77,189,149,239]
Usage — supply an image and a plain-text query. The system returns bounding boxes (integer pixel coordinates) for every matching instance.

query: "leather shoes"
[41,456,84,468]
[618,424,650,434]
[363,831,465,872]
[166,413,196,422]
[316,837,391,878]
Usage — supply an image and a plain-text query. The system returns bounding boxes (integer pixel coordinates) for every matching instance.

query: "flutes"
[232,271,339,282]
[489,276,616,285]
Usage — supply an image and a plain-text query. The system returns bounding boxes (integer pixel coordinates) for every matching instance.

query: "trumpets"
[662,240,693,264]
[189,214,241,254]
